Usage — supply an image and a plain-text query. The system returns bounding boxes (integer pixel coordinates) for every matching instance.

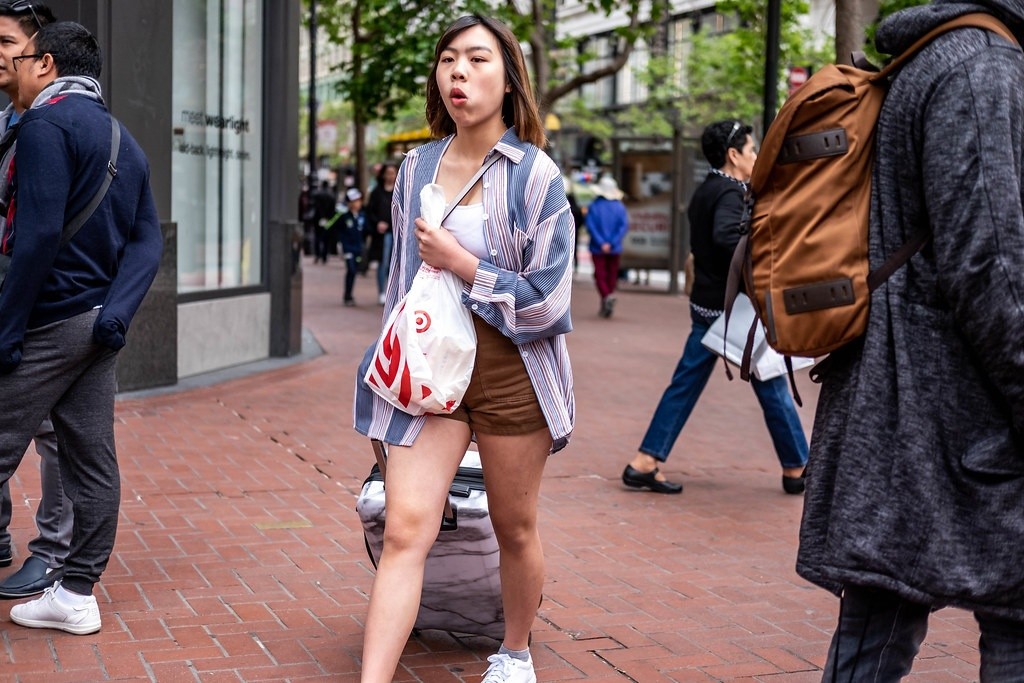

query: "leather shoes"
[782,464,807,494]
[623,464,682,493]
[0,556,66,597]
[0,549,12,566]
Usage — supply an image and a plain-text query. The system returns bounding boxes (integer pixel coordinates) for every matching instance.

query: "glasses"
[10,0,42,29]
[728,121,745,146]
[13,53,64,72]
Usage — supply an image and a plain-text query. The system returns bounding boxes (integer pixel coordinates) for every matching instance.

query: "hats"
[345,188,362,201]
[591,177,624,201]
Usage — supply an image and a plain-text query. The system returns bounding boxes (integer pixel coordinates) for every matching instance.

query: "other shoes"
[378,293,387,305]
[600,294,616,318]
[343,295,357,309]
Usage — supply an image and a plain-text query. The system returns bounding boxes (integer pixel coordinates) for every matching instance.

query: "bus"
[385,111,606,174]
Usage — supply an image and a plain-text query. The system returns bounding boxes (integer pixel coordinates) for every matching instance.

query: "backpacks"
[738,16,1020,359]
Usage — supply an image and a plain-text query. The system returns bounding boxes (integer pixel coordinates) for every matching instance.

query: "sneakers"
[481,654,537,683]
[10,580,101,635]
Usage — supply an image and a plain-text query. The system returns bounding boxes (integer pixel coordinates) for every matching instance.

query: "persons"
[796,0,1024,683]
[566,194,585,267]
[357,16,574,682]
[621,122,811,493]
[0,0,57,143]
[0,22,160,633]
[313,181,336,263]
[341,189,364,306]
[585,178,627,318]
[364,165,397,306]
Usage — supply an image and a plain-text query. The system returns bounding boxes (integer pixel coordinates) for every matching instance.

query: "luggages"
[357,437,543,647]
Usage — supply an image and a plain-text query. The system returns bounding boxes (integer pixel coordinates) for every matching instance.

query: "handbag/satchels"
[753,341,815,382]
[700,292,770,376]
[364,182,477,416]
